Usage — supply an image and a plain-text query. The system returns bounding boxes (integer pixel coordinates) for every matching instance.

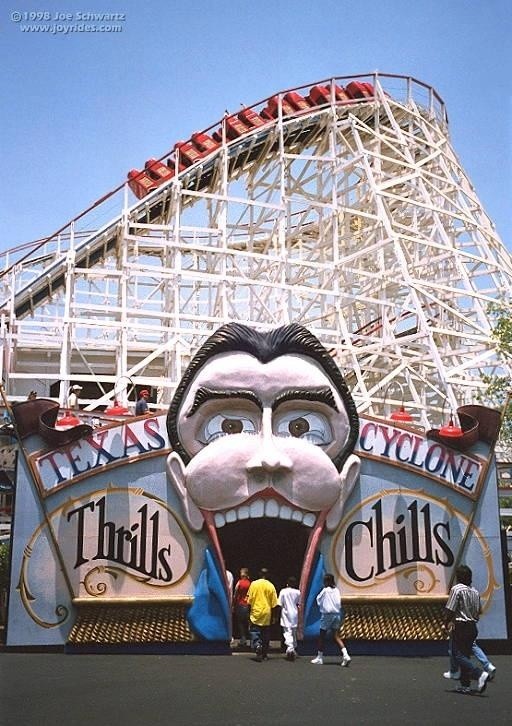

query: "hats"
[72,385,82,390]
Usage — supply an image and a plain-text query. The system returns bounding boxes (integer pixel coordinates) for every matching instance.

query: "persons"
[167,323,360,643]
[310,573,351,667]
[279,575,302,661]
[67,384,83,411]
[244,567,278,662]
[221,566,250,651]
[27,391,37,401]
[135,389,150,416]
[441,565,497,693]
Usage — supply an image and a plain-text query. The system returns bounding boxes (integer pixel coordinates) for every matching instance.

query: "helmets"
[140,390,149,397]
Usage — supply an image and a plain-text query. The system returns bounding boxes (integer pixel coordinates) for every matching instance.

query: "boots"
[251,645,268,662]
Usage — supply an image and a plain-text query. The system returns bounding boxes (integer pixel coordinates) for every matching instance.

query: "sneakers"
[311,658,323,665]
[476,663,496,693]
[287,650,296,660]
[340,656,351,666]
[456,686,472,694]
[443,671,462,680]
